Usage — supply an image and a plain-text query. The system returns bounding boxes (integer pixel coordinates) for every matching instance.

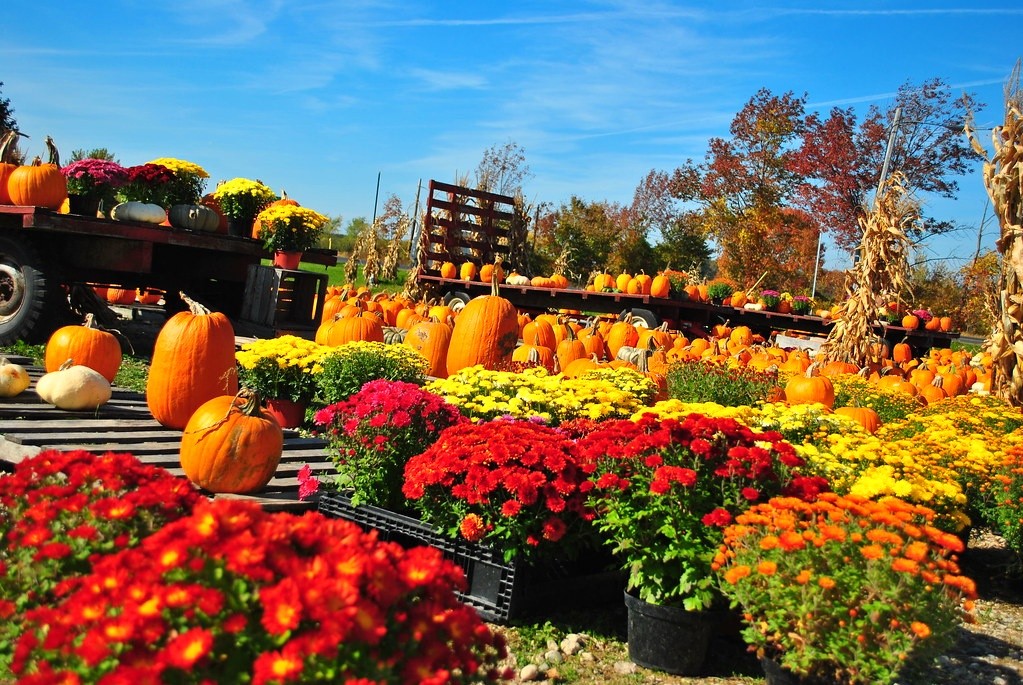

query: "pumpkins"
[0,356,31,397]
[0,130,300,233]
[87,283,163,304]
[313,259,991,434]
[45,313,122,388]
[146,291,238,431]
[35,359,111,411]
[179,388,286,497]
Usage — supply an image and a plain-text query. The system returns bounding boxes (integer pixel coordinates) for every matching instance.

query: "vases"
[67,194,100,218]
[227,215,253,239]
[778,302,790,314]
[622,586,719,677]
[796,309,804,316]
[917,319,926,330]
[274,249,304,271]
[264,398,306,428]
[948,525,973,550]
[890,319,903,327]
[766,305,775,313]
[761,656,825,685]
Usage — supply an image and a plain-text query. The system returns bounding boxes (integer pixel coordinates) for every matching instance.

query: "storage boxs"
[317,490,624,623]
[242,263,329,331]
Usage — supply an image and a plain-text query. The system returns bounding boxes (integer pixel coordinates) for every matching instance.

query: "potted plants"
[668,274,687,301]
[708,282,735,306]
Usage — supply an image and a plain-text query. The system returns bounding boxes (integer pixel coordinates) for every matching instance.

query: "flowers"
[913,310,933,322]
[792,296,811,310]
[0,331,1023,685]
[778,291,793,304]
[58,157,331,252]
[760,290,780,307]
[885,302,906,322]
